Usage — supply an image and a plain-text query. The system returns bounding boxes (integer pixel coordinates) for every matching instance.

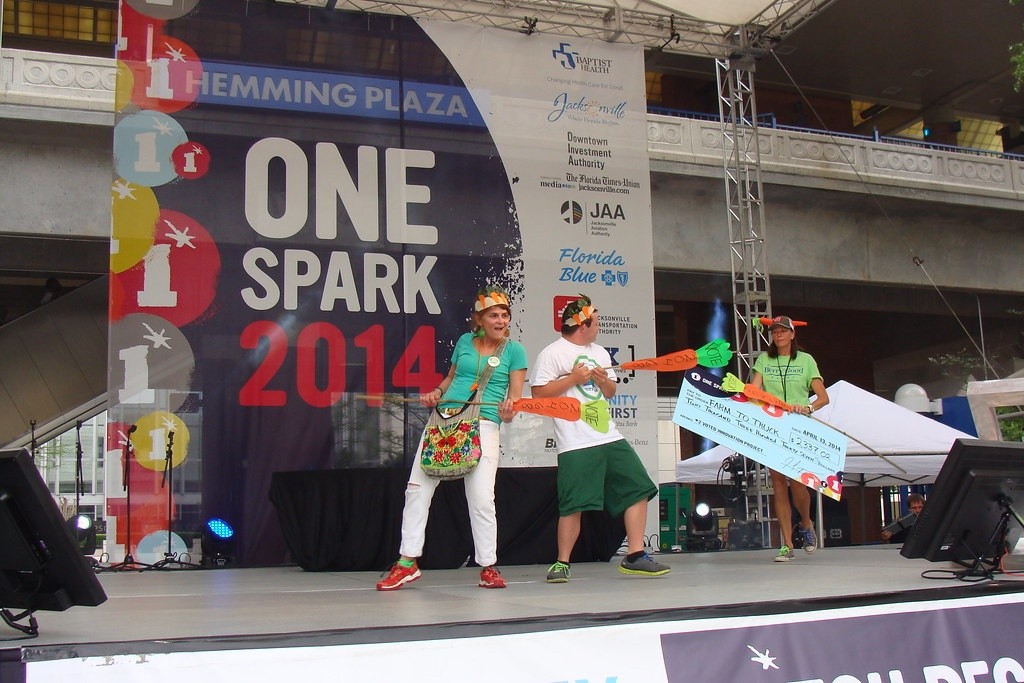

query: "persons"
[377,293,527,591]
[881,494,925,544]
[752,316,829,562]
[529,301,671,583]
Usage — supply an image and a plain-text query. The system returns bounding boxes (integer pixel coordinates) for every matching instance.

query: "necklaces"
[469,337,504,391]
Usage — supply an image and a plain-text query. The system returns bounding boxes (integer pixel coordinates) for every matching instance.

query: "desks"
[268,466,627,577]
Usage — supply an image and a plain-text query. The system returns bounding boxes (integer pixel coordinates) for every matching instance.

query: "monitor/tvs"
[899,438,1024,562]
[0,447,108,614]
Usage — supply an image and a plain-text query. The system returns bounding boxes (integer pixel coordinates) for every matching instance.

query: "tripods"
[95,425,207,573]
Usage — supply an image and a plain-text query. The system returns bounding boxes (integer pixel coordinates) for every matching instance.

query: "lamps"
[686,502,719,538]
[202,516,235,556]
[67,512,96,556]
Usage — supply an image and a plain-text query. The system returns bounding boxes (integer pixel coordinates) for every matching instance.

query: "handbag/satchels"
[421,397,483,481]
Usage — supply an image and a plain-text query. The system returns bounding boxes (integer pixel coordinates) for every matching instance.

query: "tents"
[674,380,979,549]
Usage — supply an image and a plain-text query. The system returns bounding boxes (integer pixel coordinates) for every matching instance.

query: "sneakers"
[376,558,423,591]
[799,523,817,551]
[619,552,672,575]
[479,566,507,588]
[774,545,795,562]
[546,560,571,582]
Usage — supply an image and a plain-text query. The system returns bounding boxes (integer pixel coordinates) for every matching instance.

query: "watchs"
[806,405,815,413]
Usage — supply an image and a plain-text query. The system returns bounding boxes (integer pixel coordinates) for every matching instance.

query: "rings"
[499,410,502,412]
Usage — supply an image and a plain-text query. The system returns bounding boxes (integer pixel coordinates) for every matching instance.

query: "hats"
[767,317,794,331]
